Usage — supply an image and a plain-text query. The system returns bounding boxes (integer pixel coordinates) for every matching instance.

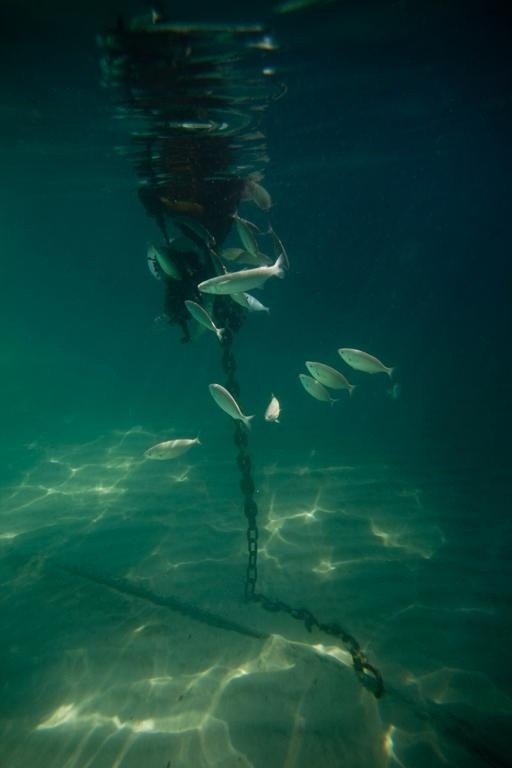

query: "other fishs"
[143,429,206,462]
